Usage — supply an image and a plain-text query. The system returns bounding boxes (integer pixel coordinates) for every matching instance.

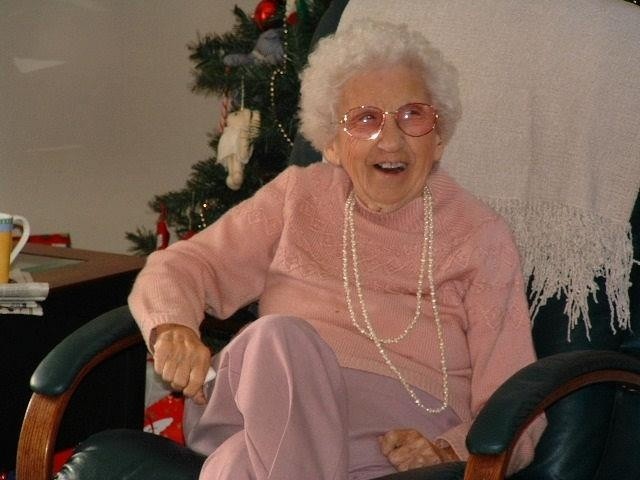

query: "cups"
[0,213,32,282]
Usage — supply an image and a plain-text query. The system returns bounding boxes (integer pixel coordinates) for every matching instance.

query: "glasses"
[338,104,439,139]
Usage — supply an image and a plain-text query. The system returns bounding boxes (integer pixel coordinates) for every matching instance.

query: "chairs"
[16,0,638,478]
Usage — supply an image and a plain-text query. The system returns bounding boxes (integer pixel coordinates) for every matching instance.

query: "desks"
[1,240,146,480]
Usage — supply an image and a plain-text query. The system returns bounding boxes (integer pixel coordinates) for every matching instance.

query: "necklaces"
[341,183,449,413]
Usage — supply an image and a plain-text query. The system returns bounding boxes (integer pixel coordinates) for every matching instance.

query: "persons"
[127,21,549,480]
